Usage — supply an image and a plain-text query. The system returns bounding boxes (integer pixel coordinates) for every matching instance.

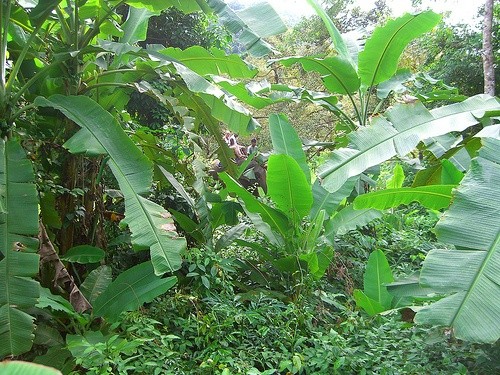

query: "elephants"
[209,155,268,200]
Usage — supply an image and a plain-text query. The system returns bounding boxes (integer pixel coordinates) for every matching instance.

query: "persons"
[218,130,246,167]
[247,138,268,168]
